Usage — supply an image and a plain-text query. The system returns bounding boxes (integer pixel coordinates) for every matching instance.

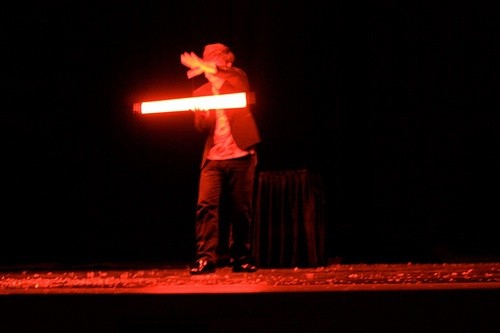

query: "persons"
[181,44,260,274]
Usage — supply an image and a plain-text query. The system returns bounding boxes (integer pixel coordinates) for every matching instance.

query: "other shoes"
[232,258,258,272]
[192,260,215,275]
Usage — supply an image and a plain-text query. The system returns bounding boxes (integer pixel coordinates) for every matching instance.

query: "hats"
[203,43,235,65]
